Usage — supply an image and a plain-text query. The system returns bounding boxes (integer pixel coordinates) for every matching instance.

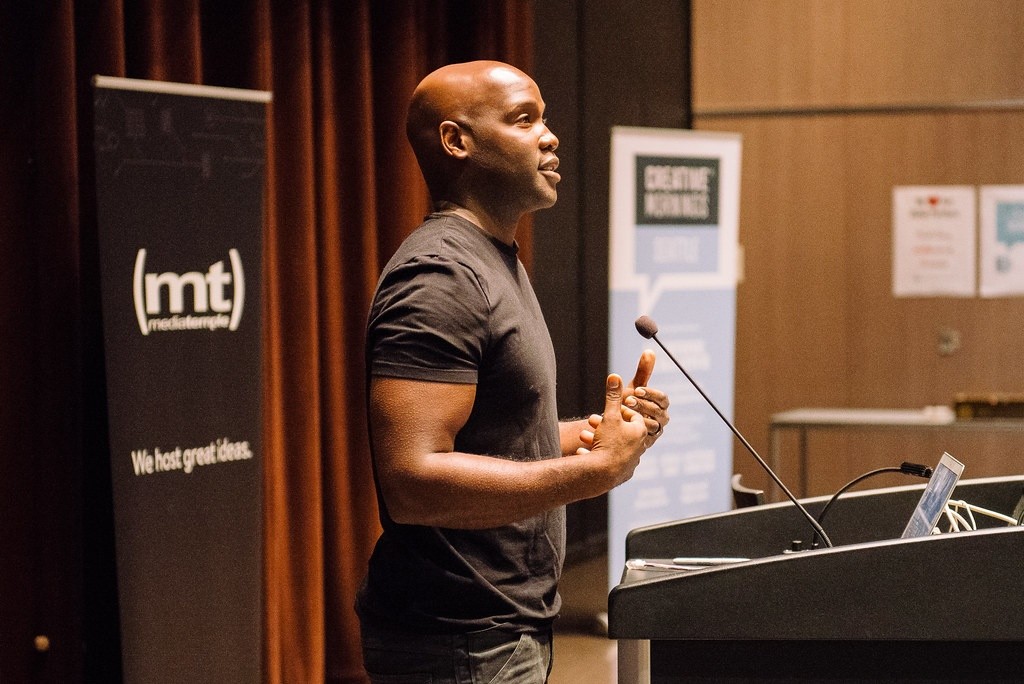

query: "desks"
[766,407,1024,506]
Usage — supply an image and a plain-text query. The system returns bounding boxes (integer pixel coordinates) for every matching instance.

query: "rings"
[648,423,661,436]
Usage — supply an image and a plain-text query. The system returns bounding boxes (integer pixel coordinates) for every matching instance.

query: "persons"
[354,60,668,684]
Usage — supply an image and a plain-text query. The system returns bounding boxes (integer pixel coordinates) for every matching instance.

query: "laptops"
[902,451,965,540]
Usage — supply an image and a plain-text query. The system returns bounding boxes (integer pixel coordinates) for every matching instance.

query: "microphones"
[634,315,833,551]
[811,462,934,551]
